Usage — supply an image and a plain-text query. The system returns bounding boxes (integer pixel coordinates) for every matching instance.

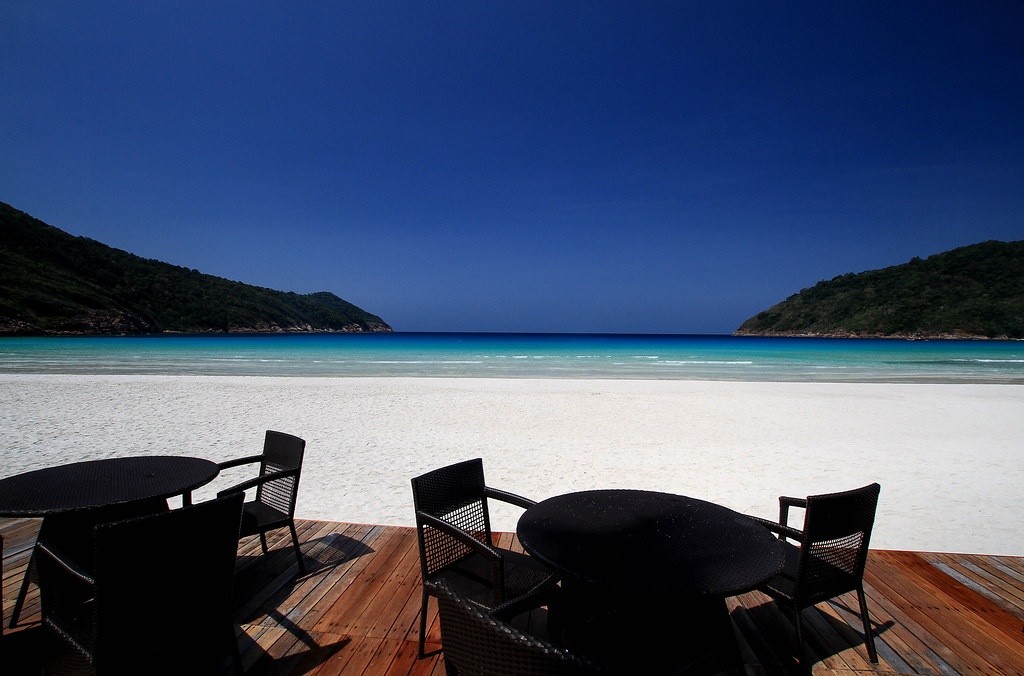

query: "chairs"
[743,483,880,676]
[411,458,538,660]
[182,430,305,577]
[434,578,602,676]
[42,493,245,676]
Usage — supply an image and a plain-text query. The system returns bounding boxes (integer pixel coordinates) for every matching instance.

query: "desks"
[1,456,220,663]
[517,489,786,676]
